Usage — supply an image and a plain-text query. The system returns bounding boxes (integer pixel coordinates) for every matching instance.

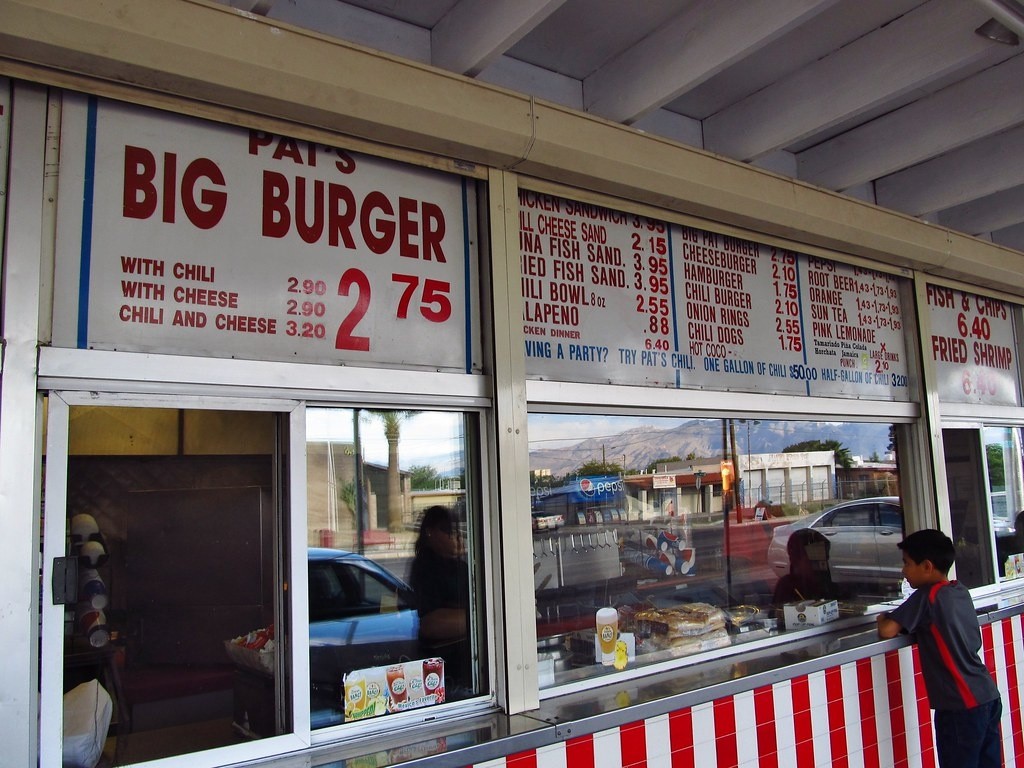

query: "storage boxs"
[224,639,289,681]
[783,600,839,630]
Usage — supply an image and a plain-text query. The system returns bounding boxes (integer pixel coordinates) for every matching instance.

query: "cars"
[530,511,564,532]
[766,493,1015,584]
[306,548,420,698]
[537,580,658,642]
[990,491,1009,517]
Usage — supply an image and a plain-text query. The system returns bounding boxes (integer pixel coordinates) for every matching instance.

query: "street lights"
[739,419,762,505]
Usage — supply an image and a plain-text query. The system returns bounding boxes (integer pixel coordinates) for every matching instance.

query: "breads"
[665,628,731,654]
[633,601,726,639]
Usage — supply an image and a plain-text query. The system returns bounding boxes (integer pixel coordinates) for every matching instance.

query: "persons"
[875,528,1003,768]
[406,501,470,639]
[768,527,832,618]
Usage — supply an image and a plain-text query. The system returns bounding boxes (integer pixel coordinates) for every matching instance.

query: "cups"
[596,607,618,666]
[385,666,407,704]
[366,675,386,716]
[78,568,108,610]
[344,675,366,711]
[79,610,109,647]
[422,660,443,695]
[623,531,696,576]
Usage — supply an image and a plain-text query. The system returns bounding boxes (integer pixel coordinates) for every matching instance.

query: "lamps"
[975,17,1019,46]
